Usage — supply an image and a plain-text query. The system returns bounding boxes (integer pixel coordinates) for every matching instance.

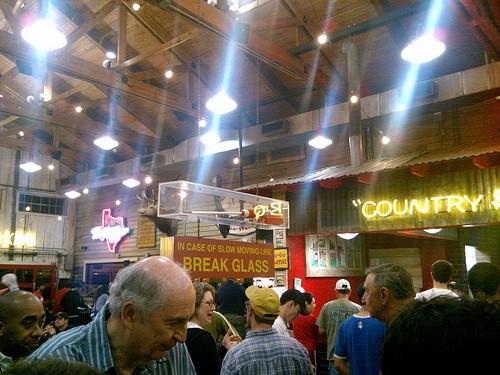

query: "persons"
[0,289,46,375]
[53,281,92,326]
[22,255,198,375]
[360,263,416,329]
[332,281,387,375]
[466,262,500,306]
[272,288,310,337]
[447,281,464,296]
[32,279,46,305]
[185,278,249,375]
[1,273,19,291]
[46,312,75,339]
[39,307,51,347]
[0,356,107,375]
[219,285,316,375]
[90,285,110,321]
[379,294,500,375]
[292,292,320,375]
[315,278,362,375]
[414,259,460,302]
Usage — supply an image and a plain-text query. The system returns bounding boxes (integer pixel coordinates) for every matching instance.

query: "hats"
[245,286,280,320]
[335,279,350,290]
[55,312,68,319]
[71,280,83,287]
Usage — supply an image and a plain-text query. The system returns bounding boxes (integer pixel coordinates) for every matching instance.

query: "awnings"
[234,143,500,190]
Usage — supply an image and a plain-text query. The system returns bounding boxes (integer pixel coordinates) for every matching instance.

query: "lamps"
[17,0,447,203]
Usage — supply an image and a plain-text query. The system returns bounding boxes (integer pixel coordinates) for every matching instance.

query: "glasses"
[200,300,216,307]
